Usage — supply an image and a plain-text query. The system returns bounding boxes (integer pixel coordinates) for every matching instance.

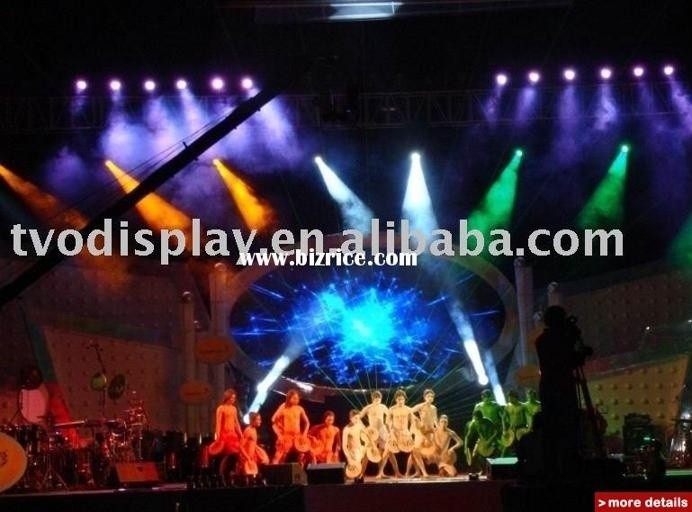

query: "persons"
[200,385,541,479]
[532,303,595,480]
